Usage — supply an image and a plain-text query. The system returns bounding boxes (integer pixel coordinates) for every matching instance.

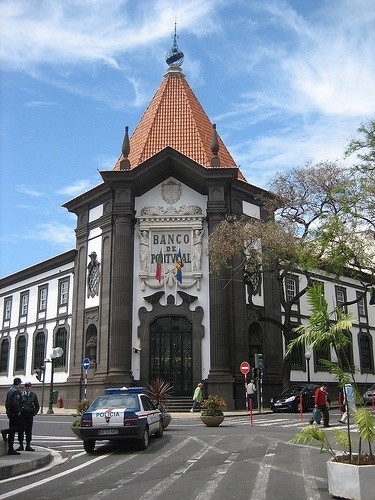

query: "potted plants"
[145,377,174,429]
[71,398,93,440]
[284,282,375,500]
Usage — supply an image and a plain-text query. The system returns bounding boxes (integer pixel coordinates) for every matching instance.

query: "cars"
[79,387,163,453]
[270,384,331,413]
[363,384,375,405]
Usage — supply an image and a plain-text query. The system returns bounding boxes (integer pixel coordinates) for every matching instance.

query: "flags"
[156,246,164,283]
[175,244,184,284]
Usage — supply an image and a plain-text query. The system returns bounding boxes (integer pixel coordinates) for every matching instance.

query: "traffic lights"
[254,353,263,369]
[36,365,45,380]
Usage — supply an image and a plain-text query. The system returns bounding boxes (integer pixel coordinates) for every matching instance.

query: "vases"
[200,416,224,426]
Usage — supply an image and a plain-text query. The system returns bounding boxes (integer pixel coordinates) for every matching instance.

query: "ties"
[27,391,28,397]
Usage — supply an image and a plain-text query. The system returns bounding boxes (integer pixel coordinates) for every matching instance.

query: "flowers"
[201,395,226,416]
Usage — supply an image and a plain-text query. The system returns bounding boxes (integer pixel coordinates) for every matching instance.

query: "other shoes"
[1,429,7,441]
[190,409,194,413]
[15,446,24,451]
[323,424,330,427]
[199,409,202,411]
[338,420,345,424]
[7,448,20,455]
[309,421,313,425]
[25,446,35,451]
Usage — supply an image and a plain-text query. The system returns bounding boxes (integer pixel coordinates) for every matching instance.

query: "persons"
[193,230,204,271]
[190,383,203,413]
[338,384,358,424]
[309,383,332,427]
[139,231,149,272]
[1,378,26,455]
[15,382,39,451]
[247,380,257,410]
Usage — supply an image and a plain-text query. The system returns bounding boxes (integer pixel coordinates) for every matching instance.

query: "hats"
[198,383,204,387]
[25,382,31,386]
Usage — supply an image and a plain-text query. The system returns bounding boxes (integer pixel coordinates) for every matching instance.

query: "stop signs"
[240,361,250,375]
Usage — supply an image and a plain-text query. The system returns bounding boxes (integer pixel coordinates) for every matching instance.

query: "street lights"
[47,347,64,414]
[305,351,312,384]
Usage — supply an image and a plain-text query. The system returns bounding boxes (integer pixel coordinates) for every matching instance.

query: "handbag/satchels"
[313,407,321,421]
